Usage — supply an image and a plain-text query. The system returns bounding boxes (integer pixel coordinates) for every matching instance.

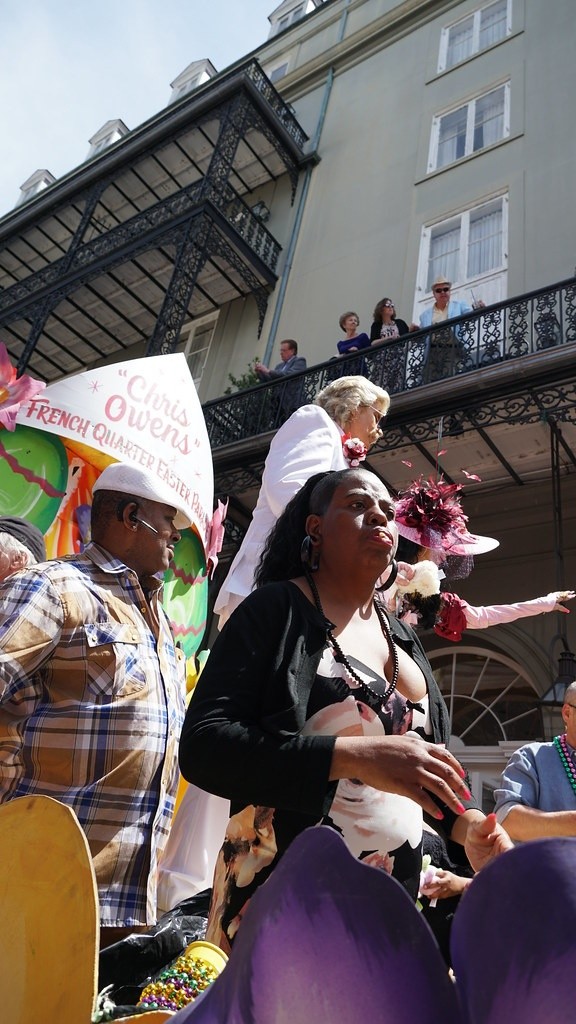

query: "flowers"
[339,433,369,471]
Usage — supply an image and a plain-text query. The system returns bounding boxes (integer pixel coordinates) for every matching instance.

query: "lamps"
[252,199,272,221]
[541,427,576,706]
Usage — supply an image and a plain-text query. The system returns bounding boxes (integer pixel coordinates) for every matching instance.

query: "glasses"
[280,348,290,353]
[434,287,450,293]
[360,403,387,427]
[384,303,395,309]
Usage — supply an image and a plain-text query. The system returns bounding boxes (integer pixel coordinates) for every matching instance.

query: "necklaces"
[305,570,399,700]
[554,735,576,798]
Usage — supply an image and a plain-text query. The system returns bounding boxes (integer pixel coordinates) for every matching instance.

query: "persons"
[255,339,308,430]
[492,681,576,842]
[415,813,476,967]
[376,476,576,628]
[211,375,390,631]
[0,462,194,945]
[409,274,487,385]
[369,297,409,393]
[178,467,471,959]
[0,514,45,584]
[337,310,370,380]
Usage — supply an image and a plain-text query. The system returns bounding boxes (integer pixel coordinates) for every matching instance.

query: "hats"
[92,460,193,530]
[394,417,500,555]
[0,516,46,564]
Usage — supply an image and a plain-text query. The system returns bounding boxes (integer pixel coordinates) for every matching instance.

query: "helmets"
[430,276,452,291]
